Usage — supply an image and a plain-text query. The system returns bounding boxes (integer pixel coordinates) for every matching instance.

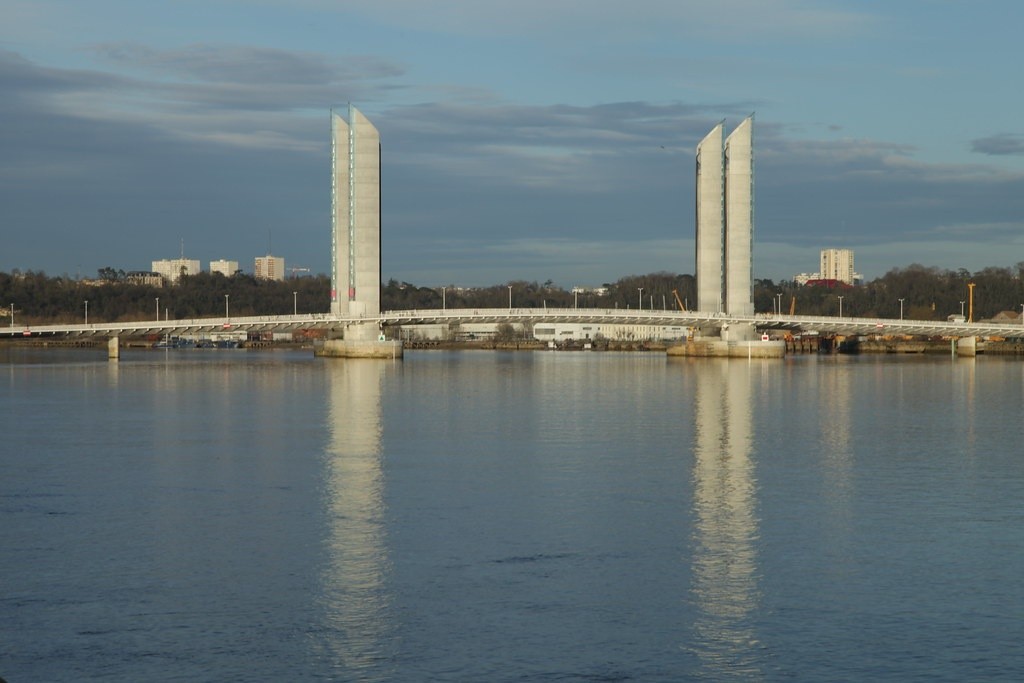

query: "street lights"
[508,286,512,308]
[293,292,297,315]
[225,295,229,317]
[442,287,446,309]
[575,290,578,308]
[838,296,844,317]
[899,299,904,320]
[85,301,88,324]
[777,294,782,315]
[1021,304,1024,324]
[156,298,159,321]
[10,304,14,327]
[960,301,965,317]
[638,288,643,310]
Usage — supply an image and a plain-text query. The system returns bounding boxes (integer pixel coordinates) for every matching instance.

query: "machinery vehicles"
[963,282,1005,342]
[783,297,794,342]
[673,290,697,341]
